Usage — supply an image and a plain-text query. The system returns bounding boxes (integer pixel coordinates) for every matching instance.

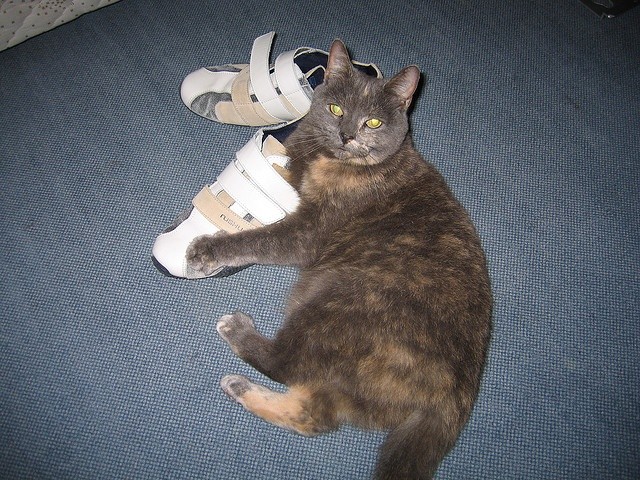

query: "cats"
[185,34,496,480]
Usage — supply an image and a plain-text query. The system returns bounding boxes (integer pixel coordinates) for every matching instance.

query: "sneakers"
[180,32,384,127]
[151,107,311,281]
